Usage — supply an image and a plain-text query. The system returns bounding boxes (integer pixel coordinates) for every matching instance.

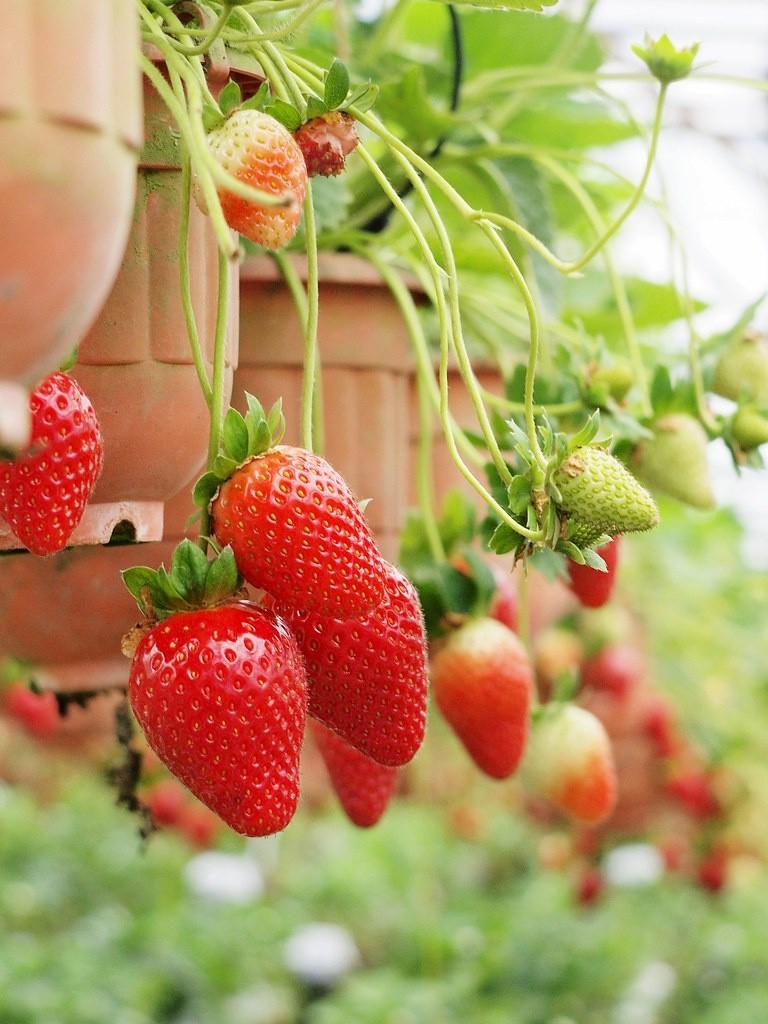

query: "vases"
[1,1,511,813]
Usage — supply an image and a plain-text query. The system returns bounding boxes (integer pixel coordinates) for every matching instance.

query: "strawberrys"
[0,102,768,910]
